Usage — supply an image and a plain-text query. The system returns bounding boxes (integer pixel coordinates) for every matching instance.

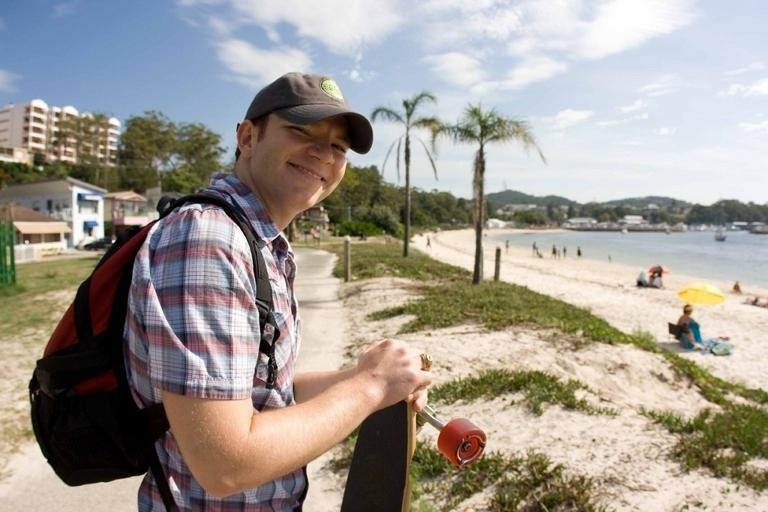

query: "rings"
[420,352,433,371]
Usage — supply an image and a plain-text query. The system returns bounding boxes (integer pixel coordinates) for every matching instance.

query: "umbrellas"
[648,264,670,274]
[676,284,724,306]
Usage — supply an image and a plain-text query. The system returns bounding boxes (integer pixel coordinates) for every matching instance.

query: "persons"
[733,280,742,293]
[742,296,768,308]
[648,272,655,288]
[653,272,663,288]
[637,271,646,286]
[678,304,705,350]
[120,71,434,511]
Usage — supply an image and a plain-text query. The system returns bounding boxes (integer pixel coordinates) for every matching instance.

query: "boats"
[716,230,727,241]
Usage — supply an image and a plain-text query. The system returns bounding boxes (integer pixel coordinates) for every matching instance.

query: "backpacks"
[28,194,281,487]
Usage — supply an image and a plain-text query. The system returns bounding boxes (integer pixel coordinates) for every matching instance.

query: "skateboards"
[340,380,487,512]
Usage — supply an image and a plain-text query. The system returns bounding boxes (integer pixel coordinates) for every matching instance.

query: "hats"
[243,72,373,155]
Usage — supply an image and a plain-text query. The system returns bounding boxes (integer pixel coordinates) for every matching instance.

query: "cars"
[84,237,115,251]
